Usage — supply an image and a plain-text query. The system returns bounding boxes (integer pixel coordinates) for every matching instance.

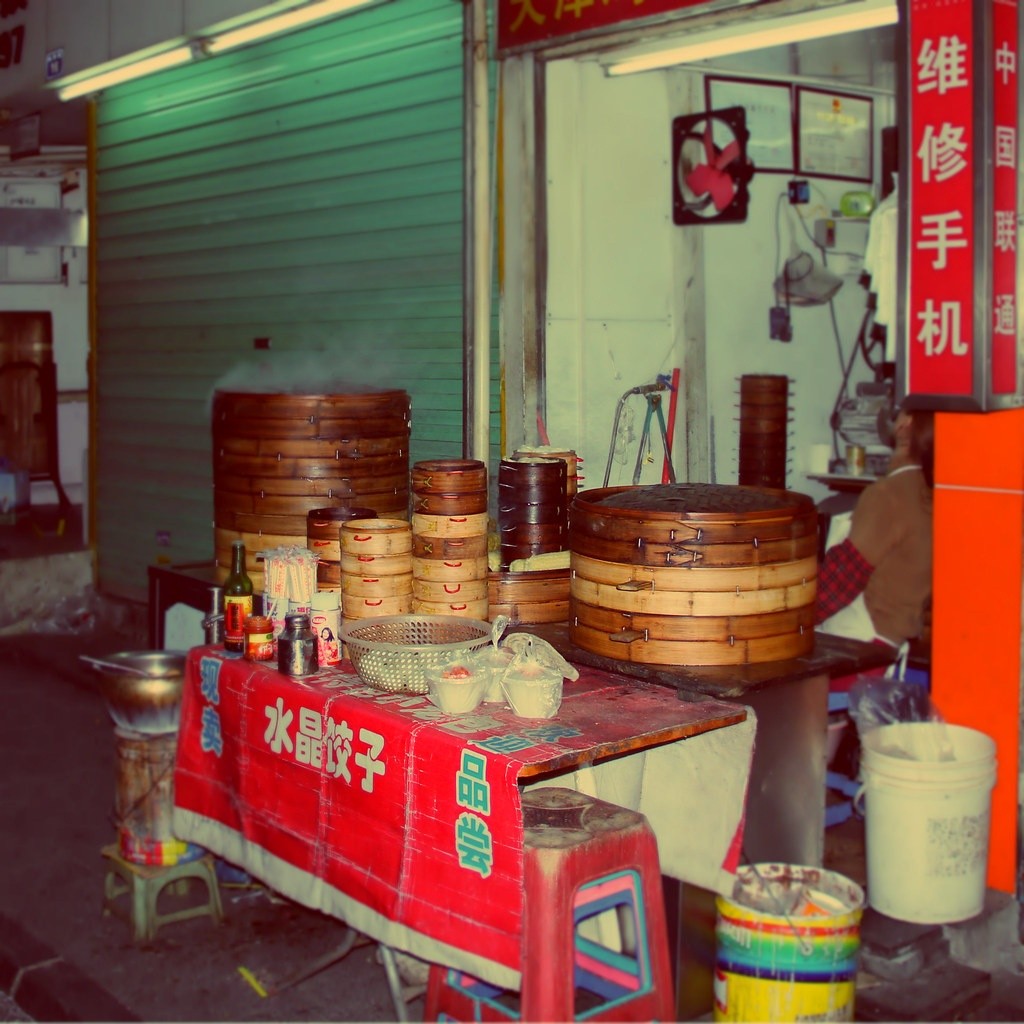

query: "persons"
[318,629,338,662]
[814,397,980,791]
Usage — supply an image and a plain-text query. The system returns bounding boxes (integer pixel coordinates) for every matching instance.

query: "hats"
[773,252,843,301]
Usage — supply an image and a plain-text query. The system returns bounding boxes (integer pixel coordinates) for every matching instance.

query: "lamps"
[600,0,902,77]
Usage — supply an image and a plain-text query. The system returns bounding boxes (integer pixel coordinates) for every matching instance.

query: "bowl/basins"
[426,665,488,712]
[483,660,508,703]
[506,669,562,717]
[94,651,190,732]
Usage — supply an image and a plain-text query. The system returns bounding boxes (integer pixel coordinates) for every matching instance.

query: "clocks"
[841,190,874,215]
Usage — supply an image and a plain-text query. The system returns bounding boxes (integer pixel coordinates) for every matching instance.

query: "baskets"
[338,614,494,695]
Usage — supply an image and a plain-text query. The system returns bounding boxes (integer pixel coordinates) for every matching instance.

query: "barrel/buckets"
[853,722,998,925]
[713,863,864,1024]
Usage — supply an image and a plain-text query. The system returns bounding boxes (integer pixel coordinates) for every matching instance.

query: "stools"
[422,786,678,1024]
[100,840,225,946]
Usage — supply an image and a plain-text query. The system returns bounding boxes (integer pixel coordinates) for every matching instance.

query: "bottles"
[223,541,254,651]
[278,615,319,675]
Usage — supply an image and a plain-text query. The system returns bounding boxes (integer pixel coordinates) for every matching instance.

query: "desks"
[172,639,757,1024]
[502,625,901,866]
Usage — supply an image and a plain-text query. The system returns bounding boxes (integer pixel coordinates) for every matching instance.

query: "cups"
[242,616,273,661]
[846,446,865,475]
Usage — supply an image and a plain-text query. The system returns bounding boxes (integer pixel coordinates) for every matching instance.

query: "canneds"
[846,444,865,477]
[243,615,274,661]
